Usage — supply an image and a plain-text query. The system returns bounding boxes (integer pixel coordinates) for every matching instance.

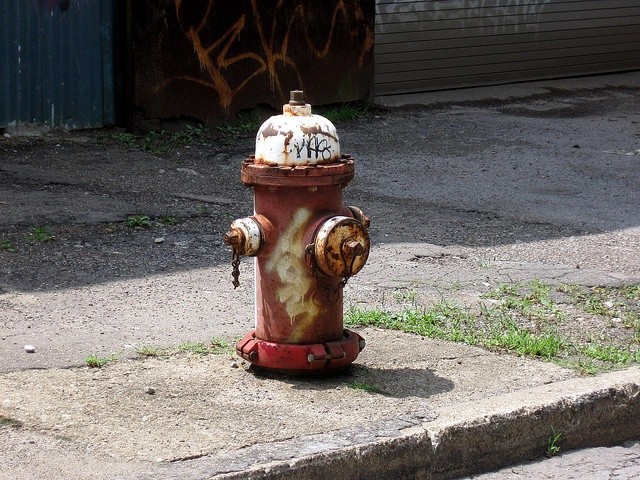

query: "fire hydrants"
[222,89,372,378]
